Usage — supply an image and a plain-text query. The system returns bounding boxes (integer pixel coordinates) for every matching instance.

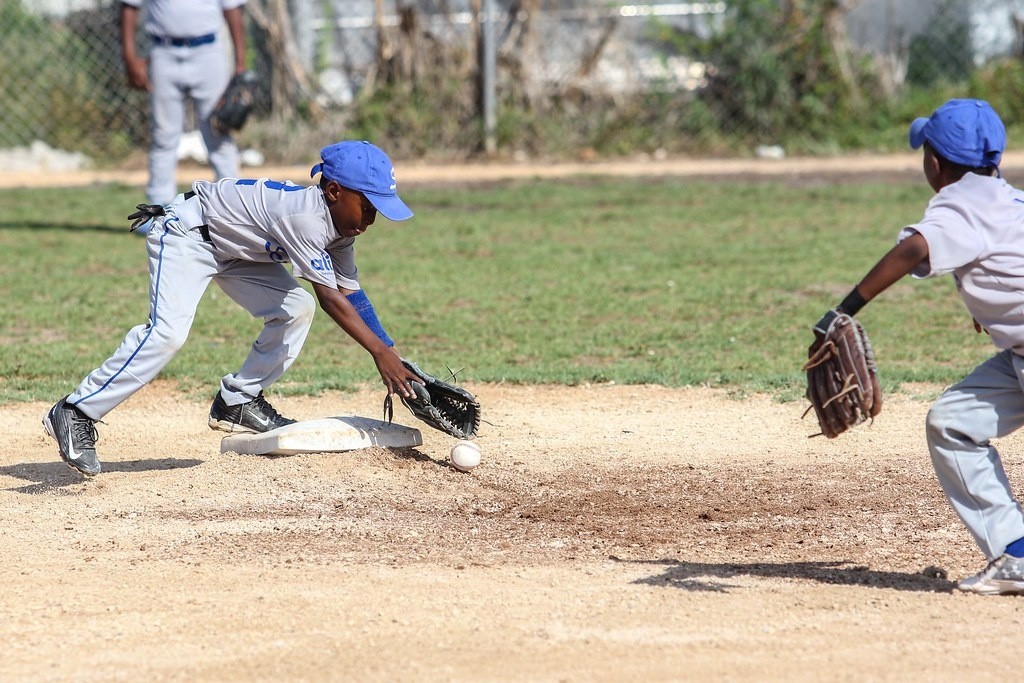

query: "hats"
[310,141,414,222]
[910,99,1006,178]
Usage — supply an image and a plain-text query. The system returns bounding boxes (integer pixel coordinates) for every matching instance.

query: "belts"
[184,191,217,249]
[154,35,214,46]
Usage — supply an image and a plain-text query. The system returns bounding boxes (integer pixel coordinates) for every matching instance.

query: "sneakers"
[958,552,1024,595]
[42,394,109,478]
[208,389,297,434]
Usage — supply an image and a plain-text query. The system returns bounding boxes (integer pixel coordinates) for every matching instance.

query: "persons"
[809,99,1024,594]
[120,0,246,236]
[40,139,426,476]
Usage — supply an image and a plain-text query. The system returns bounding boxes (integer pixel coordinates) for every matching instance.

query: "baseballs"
[449,440,483,473]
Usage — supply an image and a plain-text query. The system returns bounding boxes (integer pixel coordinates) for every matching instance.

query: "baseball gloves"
[382,356,482,441]
[802,307,883,440]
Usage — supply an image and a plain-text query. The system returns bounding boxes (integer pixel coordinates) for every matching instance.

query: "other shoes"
[136,217,153,233]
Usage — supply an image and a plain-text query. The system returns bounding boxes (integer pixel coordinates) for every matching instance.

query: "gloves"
[127,204,166,232]
